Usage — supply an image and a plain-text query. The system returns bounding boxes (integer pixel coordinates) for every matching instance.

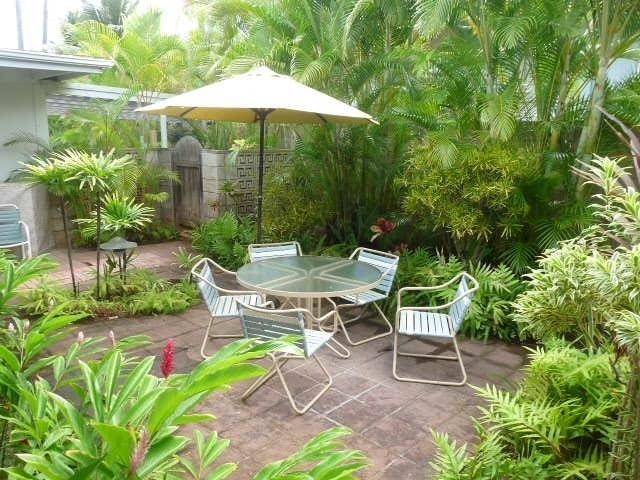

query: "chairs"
[393,270,479,387]
[191,241,400,416]
[0,203,32,260]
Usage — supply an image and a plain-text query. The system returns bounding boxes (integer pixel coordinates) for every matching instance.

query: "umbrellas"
[132,65,382,261]
